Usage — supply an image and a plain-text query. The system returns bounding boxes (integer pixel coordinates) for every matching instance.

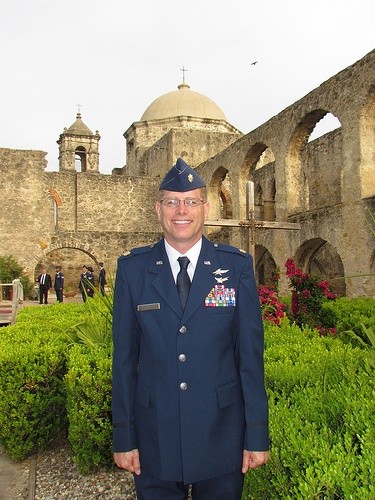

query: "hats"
[158,157,207,192]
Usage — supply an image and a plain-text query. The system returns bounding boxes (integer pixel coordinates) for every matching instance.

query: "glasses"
[158,196,205,207]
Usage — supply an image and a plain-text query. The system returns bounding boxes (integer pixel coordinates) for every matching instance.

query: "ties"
[176,256,193,312]
[42,275,44,284]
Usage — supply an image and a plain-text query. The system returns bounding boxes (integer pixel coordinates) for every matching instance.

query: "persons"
[112,158,269,500]
[98,261,107,297]
[54,266,64,303]
[78,266,94,303]
[38,268,52,304]
